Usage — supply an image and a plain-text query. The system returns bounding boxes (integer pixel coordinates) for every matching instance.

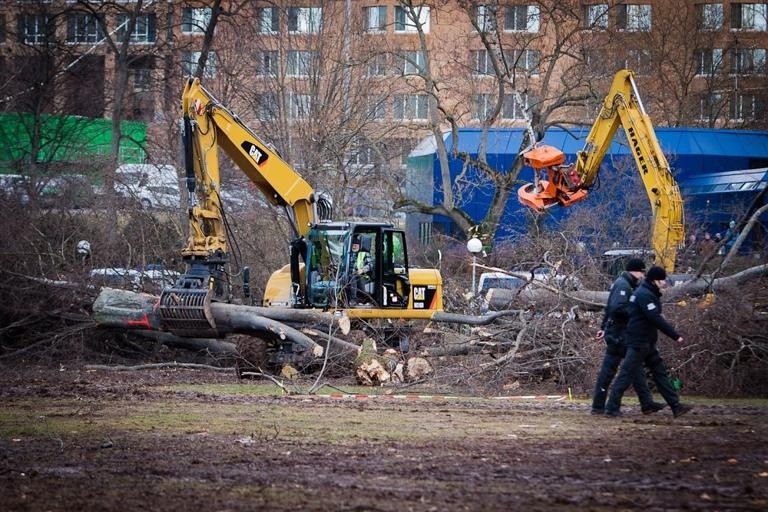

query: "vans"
[477,271,584,300]
[88,267,180,294]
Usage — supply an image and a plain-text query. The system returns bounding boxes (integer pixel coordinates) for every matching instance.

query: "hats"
[648,267,665,280]
[627,259,646,271]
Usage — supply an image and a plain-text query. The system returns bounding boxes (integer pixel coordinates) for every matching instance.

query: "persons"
[685,221,740,255]
[591,258,668,415]
[603,266,694,419]
[349,238,373,306]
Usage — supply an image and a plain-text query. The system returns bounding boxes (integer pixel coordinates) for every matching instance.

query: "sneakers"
[591,401,694,418]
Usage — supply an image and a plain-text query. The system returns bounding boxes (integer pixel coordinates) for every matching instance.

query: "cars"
[0,161,285,219]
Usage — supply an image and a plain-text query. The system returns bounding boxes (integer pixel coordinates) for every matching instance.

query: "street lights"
[467,234,483,298]
[77,241,90,274]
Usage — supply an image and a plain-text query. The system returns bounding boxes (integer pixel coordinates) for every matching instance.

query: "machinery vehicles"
[156,77,443,351]
[518,68,717,327]
